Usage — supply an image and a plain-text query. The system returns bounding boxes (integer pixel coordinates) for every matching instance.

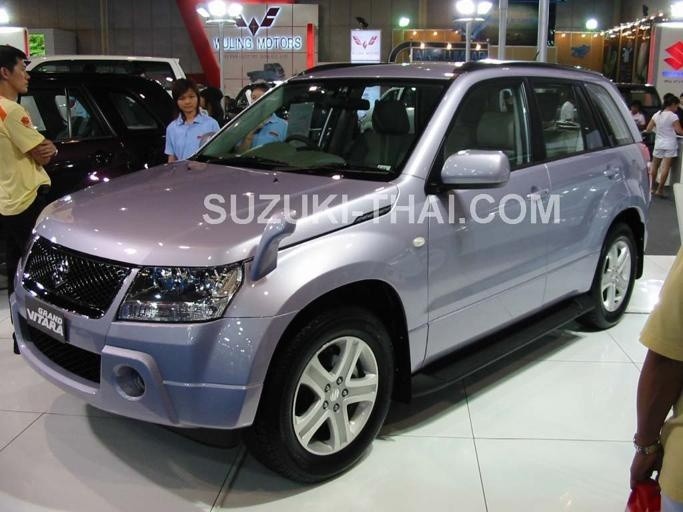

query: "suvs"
[8,60,655,485]
[13,70,181,215]
[23,55,187,102]
[612,81,664,161]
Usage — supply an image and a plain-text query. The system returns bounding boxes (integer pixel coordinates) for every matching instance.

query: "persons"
[0,44,58,354]
[164,79,288,164]
[560,97,577,122]
[627,91,683,199]
[631,243,683,512]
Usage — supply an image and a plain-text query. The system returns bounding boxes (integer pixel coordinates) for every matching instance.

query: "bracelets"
[633,434,660,455]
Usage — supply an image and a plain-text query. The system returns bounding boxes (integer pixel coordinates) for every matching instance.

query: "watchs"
[54,149,58,157]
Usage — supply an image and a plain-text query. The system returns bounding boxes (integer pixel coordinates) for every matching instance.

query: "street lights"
[196,0,243,116]
[452,0,492,62]
[398,17,410,62]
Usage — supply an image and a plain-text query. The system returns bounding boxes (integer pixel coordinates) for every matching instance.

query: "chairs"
[52,113,93,138]
[346,99,518,174]
